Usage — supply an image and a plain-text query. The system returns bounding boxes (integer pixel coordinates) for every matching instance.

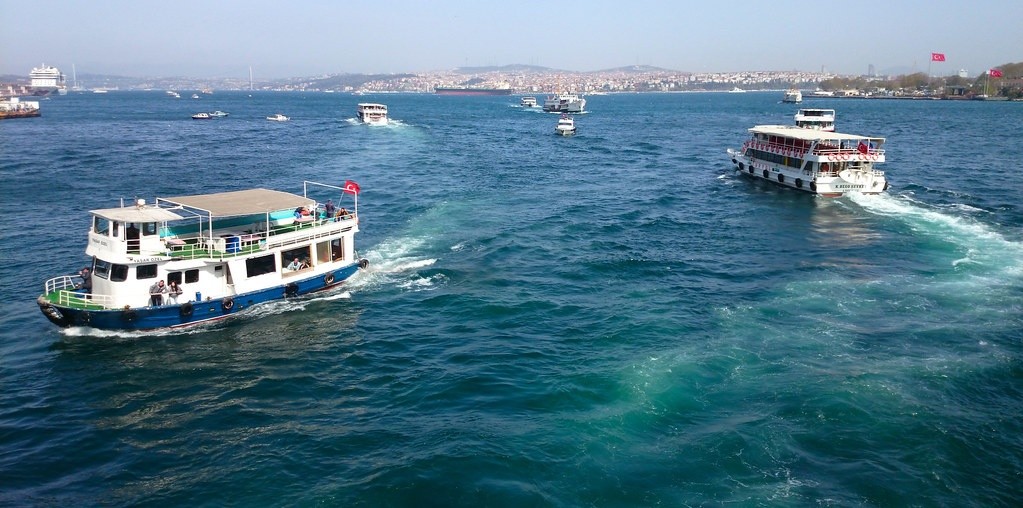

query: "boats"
[0,97,41,119]
[209,111,229,118]
[93,90,108,93]
[56,87,67,96]
[780,91,802,105]
[24,64,66,96]
[165,86,213,100]
[266,114,291,122]
[38,178,374,335]
[542,92,586,113]
[192,113,208,119]
[724,125,889,200]
[792,107,837,132]
[519,97,537,107]
[357,103,387,124]
[554,116,577,136]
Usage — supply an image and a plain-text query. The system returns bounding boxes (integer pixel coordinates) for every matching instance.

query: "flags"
[986,70,992,74]
[562,114,566,117]
[931,52,945,62]
[857,142,868,155]
[990,69,1003,76]
[343,179,360,194]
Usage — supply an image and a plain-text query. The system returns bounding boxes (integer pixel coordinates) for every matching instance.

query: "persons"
[79,267,92,299]
[750,155,756,162]
[149,280,168,307]
[287,258,308,270]
[869,143,873,149]
[805,125,811,129]
[168,281,183,304]
[840,142,844,149]
[292,200,354,221]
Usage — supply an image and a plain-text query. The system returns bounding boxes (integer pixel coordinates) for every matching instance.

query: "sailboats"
[71,65,88,91]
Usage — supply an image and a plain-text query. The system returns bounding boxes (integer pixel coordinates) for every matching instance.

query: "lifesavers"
[800,152,803,158]
[794,149,798,157]
[809,181,817,191]
[763,170,769,178]
[79,312,93,323]
[778,173,784,182]
[360,258,369,270]
[748,166,754,174]
[222,298,234,310]
[743,140,785,155]
[828,153,849,160]
[181,303,194,316]
[859,152,878,161]
[739,162,744,170]
[285,283,299,295]
[787,148,791,156]
[324,273,335,285]
[795,178,803,188]
[122,310,137,323]
